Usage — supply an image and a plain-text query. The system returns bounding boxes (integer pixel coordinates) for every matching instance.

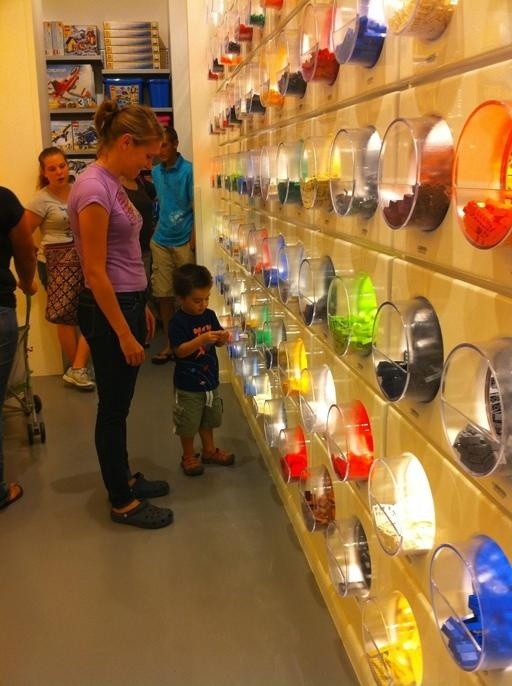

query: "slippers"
[130,471,169,499]
[109,499,174,528]
[151,349,172,365]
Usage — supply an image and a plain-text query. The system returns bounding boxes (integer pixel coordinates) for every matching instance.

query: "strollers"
[0,289,51,443]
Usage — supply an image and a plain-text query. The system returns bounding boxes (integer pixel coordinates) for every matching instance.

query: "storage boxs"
[106,78,143,106]
[146,79,170,108]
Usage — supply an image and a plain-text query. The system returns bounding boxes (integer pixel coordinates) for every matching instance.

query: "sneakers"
[201,447,235,465]
[62,366,95,392]
[179,452,204,476]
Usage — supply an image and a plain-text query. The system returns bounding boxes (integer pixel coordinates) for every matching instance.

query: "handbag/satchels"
[45,240,81,328]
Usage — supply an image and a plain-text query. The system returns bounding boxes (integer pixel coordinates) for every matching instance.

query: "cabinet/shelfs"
[46,56,105,154]
[100,70,172,113]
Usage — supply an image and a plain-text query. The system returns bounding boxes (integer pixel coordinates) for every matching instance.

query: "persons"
[20,145,101,391]
[0,183,40,509]
[66,94,176,529]
[169,263,236,475]
[113,173,157,350]
[148,123,197,365]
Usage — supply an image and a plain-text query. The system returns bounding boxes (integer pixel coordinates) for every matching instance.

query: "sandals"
[6,481,23,505]
